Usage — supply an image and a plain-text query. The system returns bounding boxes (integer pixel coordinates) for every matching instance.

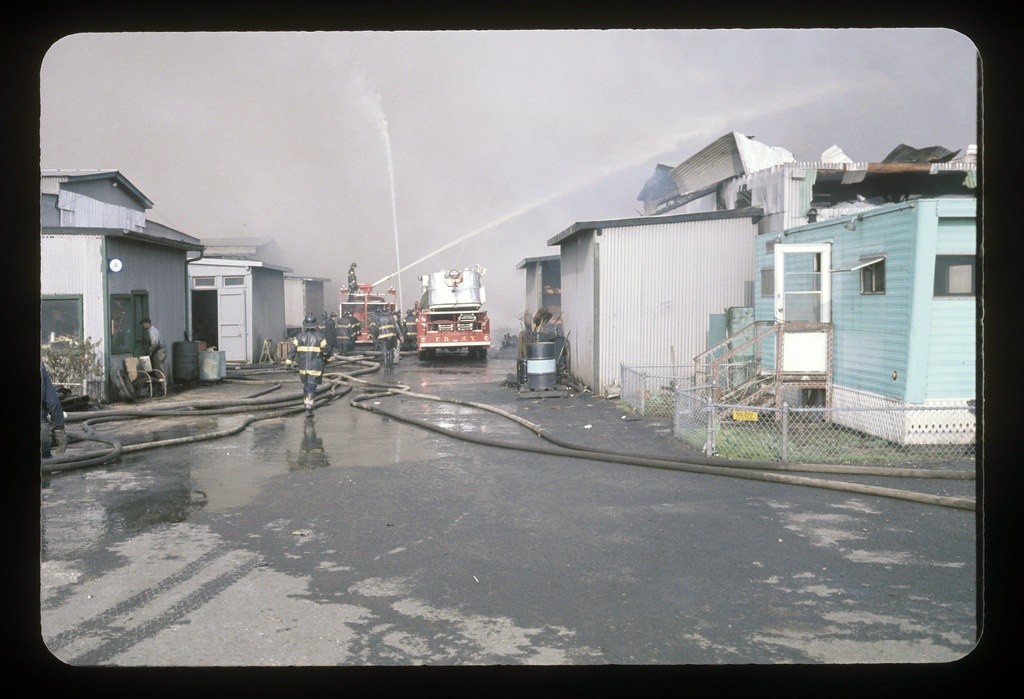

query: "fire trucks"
[338,284,397,346]
[413,266,491,361]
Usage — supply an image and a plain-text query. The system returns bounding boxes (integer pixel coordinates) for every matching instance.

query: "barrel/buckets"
[526,342,557,389]
[172,340,227,385]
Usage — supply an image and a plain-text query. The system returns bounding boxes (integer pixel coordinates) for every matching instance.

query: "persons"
[139,318,167,396]
[284,314,332,417]
[41,360,68,489]
[50,302,76,347]
[348,263,357,302]
[319,305,417,372]
[111,302,126,348]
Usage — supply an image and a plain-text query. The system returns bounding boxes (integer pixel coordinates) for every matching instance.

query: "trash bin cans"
[198,349,227,380]
[526,342,558,391]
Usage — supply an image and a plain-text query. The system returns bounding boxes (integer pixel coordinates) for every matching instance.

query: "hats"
[139,318,150,324]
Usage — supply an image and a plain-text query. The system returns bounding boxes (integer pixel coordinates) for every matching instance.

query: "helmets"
[330,312,337,316]
[406,310,411,314]
[322,312,327,316]
[351,263,356,267]
[303,315,318,329]
[376,306,390,314]
[343,311,353,316]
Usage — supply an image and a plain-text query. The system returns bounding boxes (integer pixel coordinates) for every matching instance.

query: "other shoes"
[389,363,393,369]
[306,410,314,417]
[384,369,388,371]
[155,392,164,397]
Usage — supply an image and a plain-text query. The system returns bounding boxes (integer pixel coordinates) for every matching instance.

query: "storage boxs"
[276,340,293,360]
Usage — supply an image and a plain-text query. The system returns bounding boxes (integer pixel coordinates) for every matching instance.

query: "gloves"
[51,430,69,453]
[286,365,291,370]
